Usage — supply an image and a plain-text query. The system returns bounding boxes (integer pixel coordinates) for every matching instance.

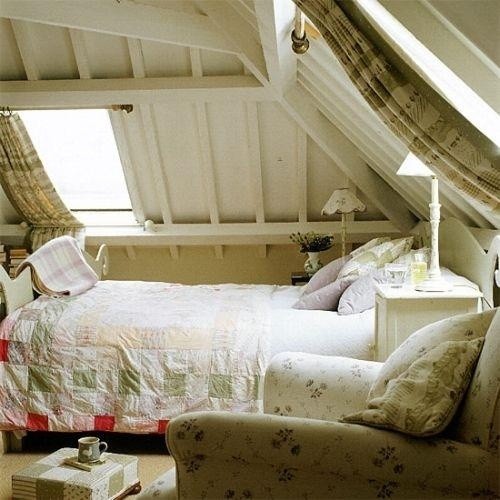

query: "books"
[0,244,29,292]
[63,453,111,473]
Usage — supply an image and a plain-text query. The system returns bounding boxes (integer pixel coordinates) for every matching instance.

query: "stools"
[11,446,141,499]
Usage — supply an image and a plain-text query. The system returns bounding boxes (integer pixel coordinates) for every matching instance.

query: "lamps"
[395,151,452,292]
[320,188,367,257]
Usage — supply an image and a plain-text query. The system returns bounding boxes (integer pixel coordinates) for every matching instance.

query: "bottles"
[411,253,428,284]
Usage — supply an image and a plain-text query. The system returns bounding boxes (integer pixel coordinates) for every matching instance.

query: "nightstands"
[371,277,484,364]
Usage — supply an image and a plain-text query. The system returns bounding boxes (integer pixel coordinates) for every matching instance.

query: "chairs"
[133,304,498,499]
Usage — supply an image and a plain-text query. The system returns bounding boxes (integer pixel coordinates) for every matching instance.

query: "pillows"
[338,247,430,315]
[336,308,496,410]
[290,236,416,311]
[338,336,486,442]
[297,235,392,299]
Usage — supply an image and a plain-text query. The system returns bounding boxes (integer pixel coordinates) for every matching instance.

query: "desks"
[289,270,312,285]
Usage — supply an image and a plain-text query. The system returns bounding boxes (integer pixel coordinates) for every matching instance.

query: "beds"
[0,214,499,458]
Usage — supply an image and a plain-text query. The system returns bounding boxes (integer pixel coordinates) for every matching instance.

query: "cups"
[77,437,109,462]
[385,263,407,289]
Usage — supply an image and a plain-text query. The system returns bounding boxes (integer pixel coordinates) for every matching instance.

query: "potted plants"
[287,231,335,276]
